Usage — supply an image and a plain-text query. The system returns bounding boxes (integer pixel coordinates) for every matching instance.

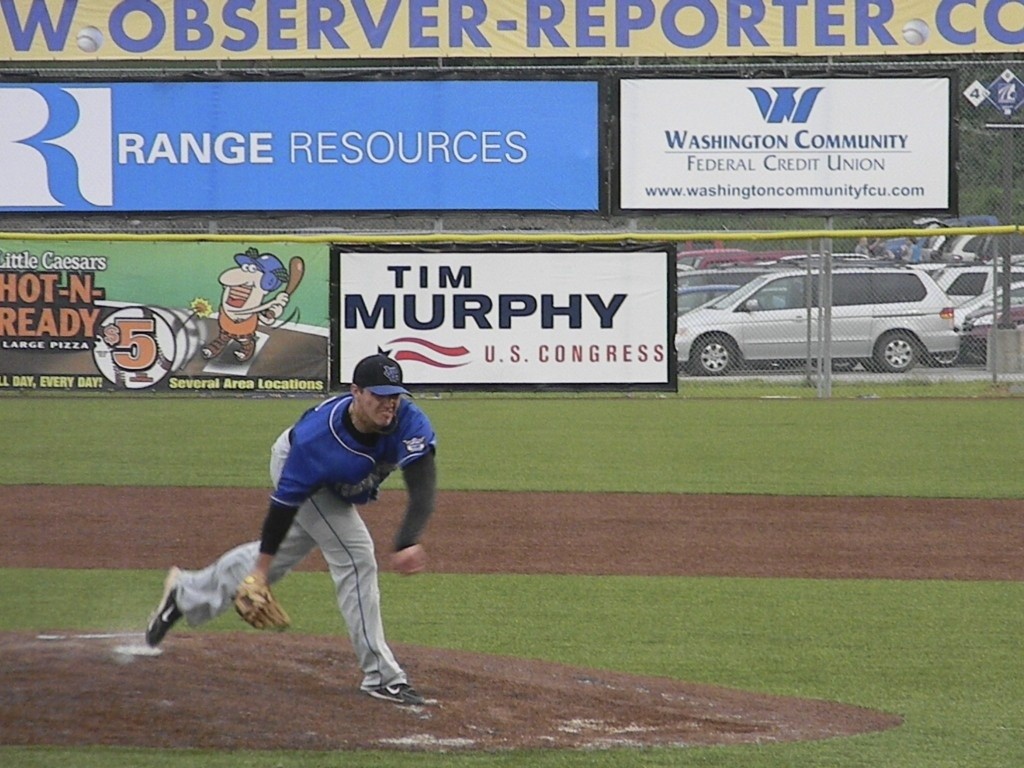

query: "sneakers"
[146,566,184,647]
[360,683,438,704]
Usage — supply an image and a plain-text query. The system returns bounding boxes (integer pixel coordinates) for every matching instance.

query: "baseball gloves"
[233,573,290,631]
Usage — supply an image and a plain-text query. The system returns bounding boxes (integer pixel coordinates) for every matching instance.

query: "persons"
[146,355,440,706]
[852,236,920,265]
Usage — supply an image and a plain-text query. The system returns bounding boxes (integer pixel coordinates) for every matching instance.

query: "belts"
[288,427,293,446]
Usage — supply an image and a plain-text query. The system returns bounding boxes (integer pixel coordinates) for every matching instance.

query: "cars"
[674,257,959,378]
[664,212,1024,378]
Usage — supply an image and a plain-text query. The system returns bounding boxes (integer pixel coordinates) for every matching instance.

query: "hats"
[354,355,412,399]
